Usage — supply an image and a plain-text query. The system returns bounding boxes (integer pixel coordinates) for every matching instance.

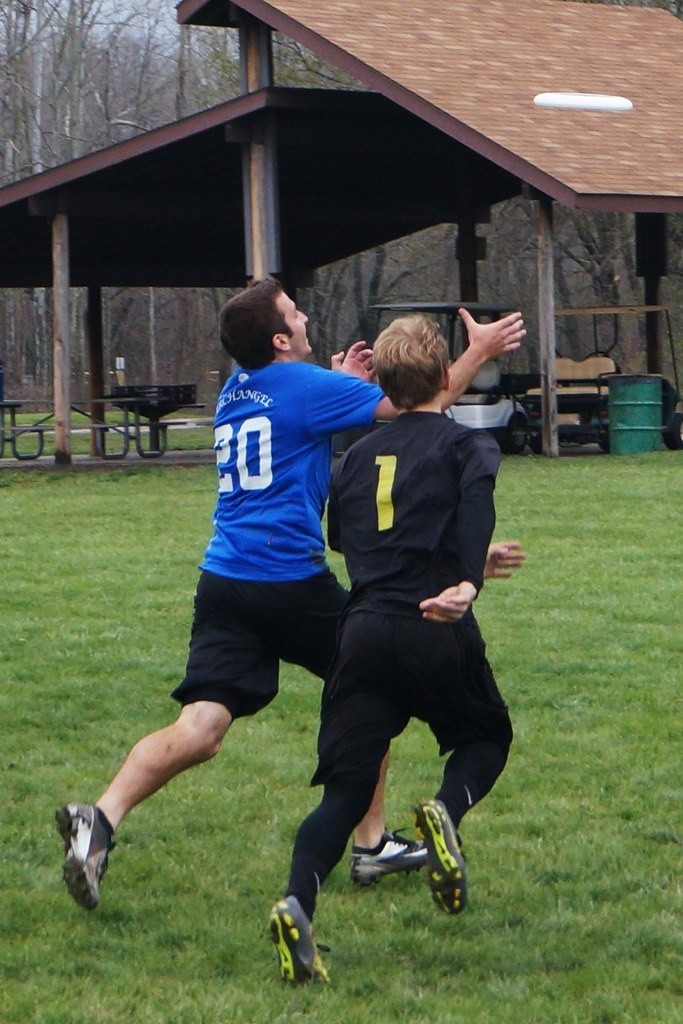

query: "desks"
[0,396,170,461]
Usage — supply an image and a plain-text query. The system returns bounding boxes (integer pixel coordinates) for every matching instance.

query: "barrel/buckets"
[608,374,663,456]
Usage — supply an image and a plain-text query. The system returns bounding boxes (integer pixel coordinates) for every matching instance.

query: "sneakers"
[350,829,429,886]
[55,802,116,912]
[412,798,469,915]
[268,896,331,988]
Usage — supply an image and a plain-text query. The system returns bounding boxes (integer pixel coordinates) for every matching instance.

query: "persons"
[268,315,526,989]
[56,279,528,910]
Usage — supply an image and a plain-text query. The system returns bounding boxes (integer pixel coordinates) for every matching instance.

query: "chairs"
[454,361,500,404]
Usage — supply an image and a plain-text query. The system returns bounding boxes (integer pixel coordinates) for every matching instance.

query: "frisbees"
[532,91,634,111]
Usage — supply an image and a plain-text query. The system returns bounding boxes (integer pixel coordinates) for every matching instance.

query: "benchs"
[0,421,188,436]
[525,357,614,403]
